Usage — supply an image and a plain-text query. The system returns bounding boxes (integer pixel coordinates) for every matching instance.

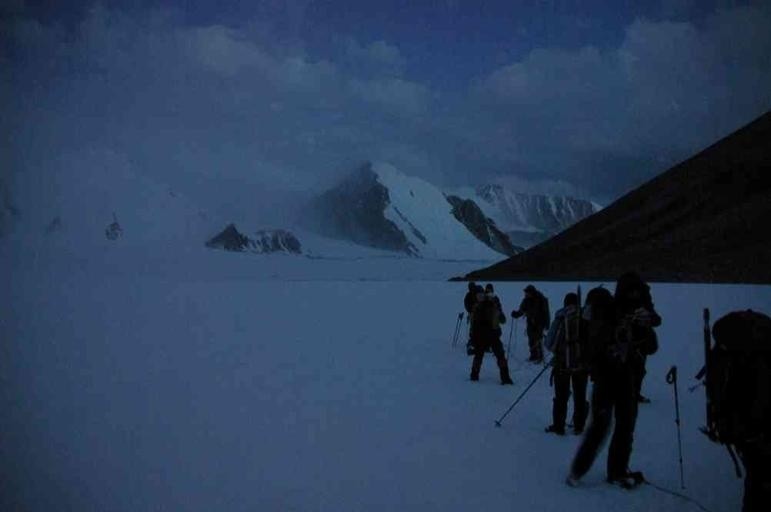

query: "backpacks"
[705,310,771,445]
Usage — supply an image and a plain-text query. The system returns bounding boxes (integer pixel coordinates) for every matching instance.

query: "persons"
[544,280,661,490]
[511,284,550,364]
[740,311,770,512]
[464,282,513,385]
